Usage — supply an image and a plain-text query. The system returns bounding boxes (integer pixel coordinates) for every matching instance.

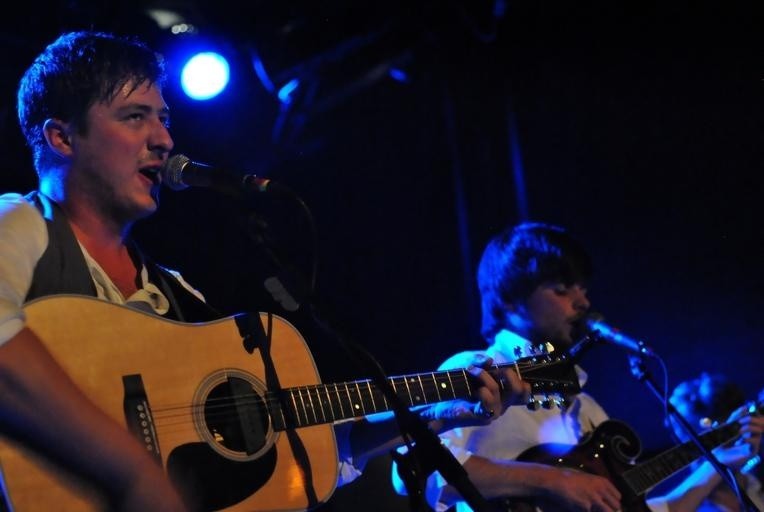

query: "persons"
[407,219,763,511]
[666,369,763,510]
[0,26,530,511]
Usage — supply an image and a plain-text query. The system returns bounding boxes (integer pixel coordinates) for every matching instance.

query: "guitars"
[493,397,762,511]
[0,292,586,511]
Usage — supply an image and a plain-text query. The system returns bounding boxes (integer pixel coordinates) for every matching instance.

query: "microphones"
[581,312,664,361]
[160,154,272,191]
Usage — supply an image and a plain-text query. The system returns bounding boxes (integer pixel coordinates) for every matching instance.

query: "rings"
[482,403,499,417]
